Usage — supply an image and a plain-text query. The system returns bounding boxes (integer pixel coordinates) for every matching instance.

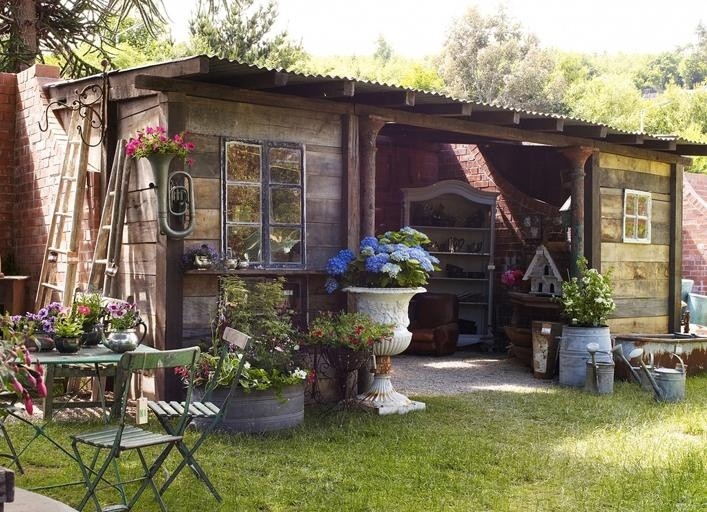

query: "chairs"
[69,343,203,512]
[402,292,460,358]
[146,325,254,504]
[32,288,131,420]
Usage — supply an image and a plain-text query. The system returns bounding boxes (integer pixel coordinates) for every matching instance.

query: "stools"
[0,389,26,475]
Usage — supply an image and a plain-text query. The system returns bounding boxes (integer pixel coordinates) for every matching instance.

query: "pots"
[502,324,534,348]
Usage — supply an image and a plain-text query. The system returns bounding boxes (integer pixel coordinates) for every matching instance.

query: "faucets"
[680,314,689,326]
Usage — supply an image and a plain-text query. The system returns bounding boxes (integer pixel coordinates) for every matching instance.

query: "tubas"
[141,151,196,240]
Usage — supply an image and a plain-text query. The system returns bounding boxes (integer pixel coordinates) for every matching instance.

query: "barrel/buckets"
[532,322,562,378]
[558,324,613,386]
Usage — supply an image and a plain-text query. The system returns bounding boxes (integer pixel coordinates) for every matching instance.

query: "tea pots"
[444,236,464,252]
[194,254,212,272]
[96,323,147,353]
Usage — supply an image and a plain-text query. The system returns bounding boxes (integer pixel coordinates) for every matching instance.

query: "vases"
[340,285,433,416]
[19,323,138,350]
[323,341,380,416]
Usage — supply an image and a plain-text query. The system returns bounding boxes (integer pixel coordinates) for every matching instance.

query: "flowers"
[0,289,141,334]
[548,254,618,327]
[124,124,198,170]
[303,306,395,346]
[176,273,311,405]
[320,225,440,295]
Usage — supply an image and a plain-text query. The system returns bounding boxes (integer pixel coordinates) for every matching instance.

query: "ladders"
[66,138,135,401]
[32,104,94,316]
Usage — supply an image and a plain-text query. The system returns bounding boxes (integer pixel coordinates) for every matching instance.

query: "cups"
[466,240,482,253]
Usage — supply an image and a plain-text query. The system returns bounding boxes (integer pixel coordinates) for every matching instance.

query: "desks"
[7,341,163,512]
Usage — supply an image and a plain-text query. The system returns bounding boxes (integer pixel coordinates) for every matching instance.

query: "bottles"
[426,240,440,253]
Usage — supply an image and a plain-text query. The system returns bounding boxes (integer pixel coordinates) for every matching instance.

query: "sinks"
[615,333,706,383]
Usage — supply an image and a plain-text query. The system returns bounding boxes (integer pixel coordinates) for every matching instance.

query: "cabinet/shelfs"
[397,177,500,351]
